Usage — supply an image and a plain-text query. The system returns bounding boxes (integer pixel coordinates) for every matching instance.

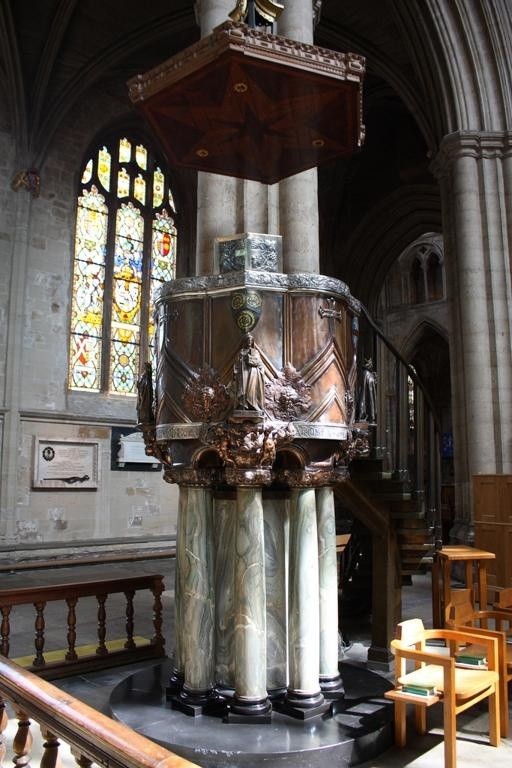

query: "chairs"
[384,586,512,765]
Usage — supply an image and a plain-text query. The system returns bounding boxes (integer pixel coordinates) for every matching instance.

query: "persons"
[359,357,380,423]
[233,330,266,410]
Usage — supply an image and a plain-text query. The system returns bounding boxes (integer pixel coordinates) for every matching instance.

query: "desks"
[434,543,495,629]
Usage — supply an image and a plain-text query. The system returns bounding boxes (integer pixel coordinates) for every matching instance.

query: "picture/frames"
[32,438,102,493]
